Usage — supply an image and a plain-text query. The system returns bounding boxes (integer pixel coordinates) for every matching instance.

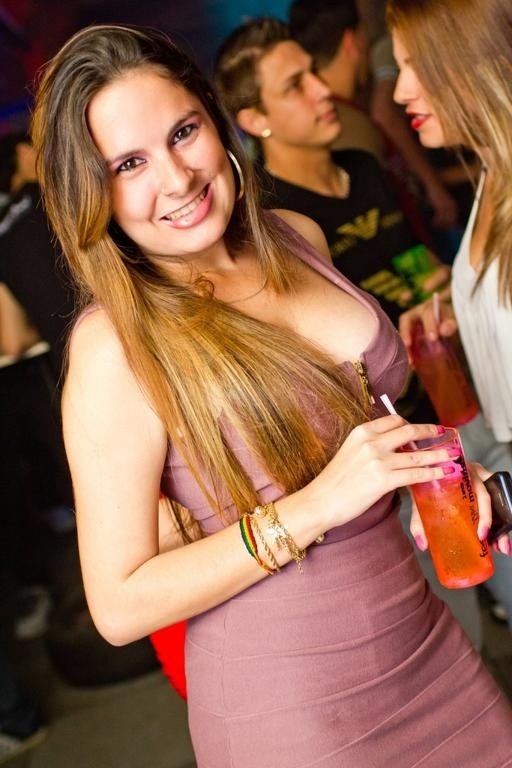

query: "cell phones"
[483,471,512,546]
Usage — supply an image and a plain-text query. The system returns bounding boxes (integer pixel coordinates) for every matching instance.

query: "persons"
[32,24,512,768]
[0,0,511,755]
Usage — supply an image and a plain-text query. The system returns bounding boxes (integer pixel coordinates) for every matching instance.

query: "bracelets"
[235,499,327,576]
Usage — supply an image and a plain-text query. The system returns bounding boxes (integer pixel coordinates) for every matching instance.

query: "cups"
[405,317,481,427]
[404,424,497,588]
